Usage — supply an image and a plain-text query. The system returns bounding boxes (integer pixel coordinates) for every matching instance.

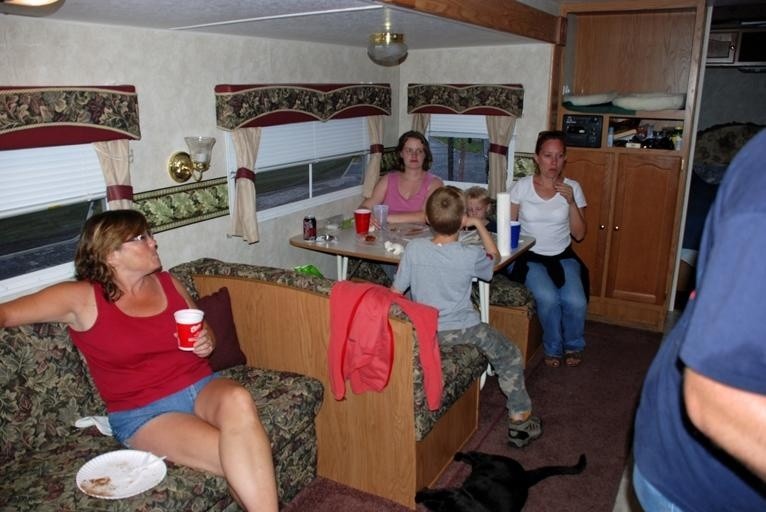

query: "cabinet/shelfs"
[702,27,764,68]
[555,2,708,334]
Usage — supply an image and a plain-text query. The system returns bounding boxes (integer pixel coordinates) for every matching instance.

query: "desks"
[286,220,538,380]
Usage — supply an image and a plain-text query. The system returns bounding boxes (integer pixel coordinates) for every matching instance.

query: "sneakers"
[507,415,544,449]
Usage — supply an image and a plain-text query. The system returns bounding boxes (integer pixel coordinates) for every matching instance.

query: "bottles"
[607,127,614,148]
[672,126,683,151]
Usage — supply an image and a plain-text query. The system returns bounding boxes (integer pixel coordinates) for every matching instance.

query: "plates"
[458,231,498,246]
[73,448,169,503]
[354,231,393,248]
[383,223,432,236]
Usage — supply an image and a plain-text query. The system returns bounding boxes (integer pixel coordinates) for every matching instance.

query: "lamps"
[363,29,409,68]
[167,131,215,186]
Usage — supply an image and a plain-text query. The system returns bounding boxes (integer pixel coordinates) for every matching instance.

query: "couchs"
[0,264,326,510]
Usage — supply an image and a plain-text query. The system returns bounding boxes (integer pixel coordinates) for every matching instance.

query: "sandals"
[544,356,561,368]
[566,351,582,367]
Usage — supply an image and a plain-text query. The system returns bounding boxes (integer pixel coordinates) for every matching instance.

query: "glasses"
[132,229,152,241]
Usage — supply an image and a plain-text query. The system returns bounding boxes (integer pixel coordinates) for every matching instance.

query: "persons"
[509,130,589,369]
[390,185,545,448]
[460,186,498,233]
[0,209,281,512]
[356,130,444,232]
[610,126,766,511]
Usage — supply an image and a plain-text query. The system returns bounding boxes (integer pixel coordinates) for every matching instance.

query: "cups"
[509,220,521,247]
[173,307,204,350]
[353,208,371,235]
[371,204,389,232]
[552,175,565,190]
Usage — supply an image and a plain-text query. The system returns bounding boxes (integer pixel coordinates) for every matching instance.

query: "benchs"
[181,252,490,512]
[345,199,550,375]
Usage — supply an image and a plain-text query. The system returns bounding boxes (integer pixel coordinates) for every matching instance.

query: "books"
[614,128,637,140]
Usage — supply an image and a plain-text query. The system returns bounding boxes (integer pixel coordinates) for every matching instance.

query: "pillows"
[193,285,247,375]
